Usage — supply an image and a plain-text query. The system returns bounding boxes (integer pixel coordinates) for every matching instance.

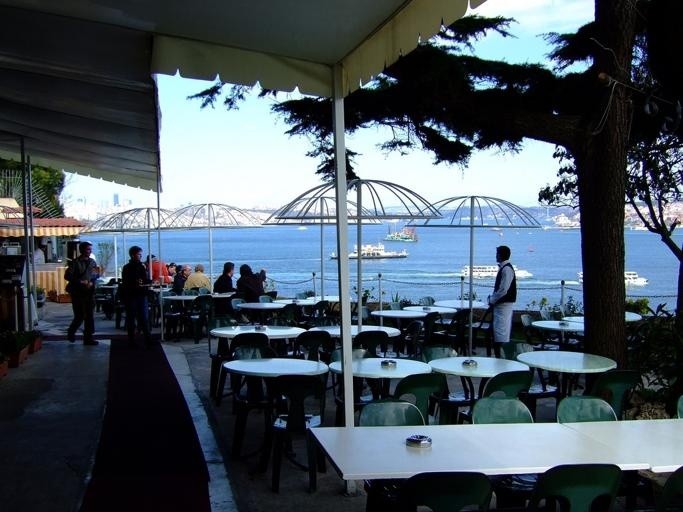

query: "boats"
[328,243,410,260]
[576,269,651,289]
[459,263,538,278]
[382,223,420,242]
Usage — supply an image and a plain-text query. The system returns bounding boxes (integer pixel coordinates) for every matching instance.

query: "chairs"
[472,397,557,511]
[677,395,683,419]
[261,375,327,494]
[657,466,683,512]
[458,370,533,425]
[376,372,443,425]
[472,397,534,424]
[331,349,381,427]
[359,401,426,511]
[229,342,279,464]
[147,285,214,344]
[397,472,494,512]
[589,369,641,421]
[421,346,469,425]
[502,339,561,423]
[527,463,622,512]
[93,278,125,329]
[207,286,643,407]
[557,396,646,511]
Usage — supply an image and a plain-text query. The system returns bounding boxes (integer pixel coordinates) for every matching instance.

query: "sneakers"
[68,334,98,346]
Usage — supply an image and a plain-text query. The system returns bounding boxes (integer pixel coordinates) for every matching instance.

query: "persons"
[122,246,266,336]
[64,242,99,345]
[19,238,48,287]
[487,247,517,359]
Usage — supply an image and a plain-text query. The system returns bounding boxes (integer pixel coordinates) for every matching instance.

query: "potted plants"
[0,330,43,379]
[31,284,45,308]
